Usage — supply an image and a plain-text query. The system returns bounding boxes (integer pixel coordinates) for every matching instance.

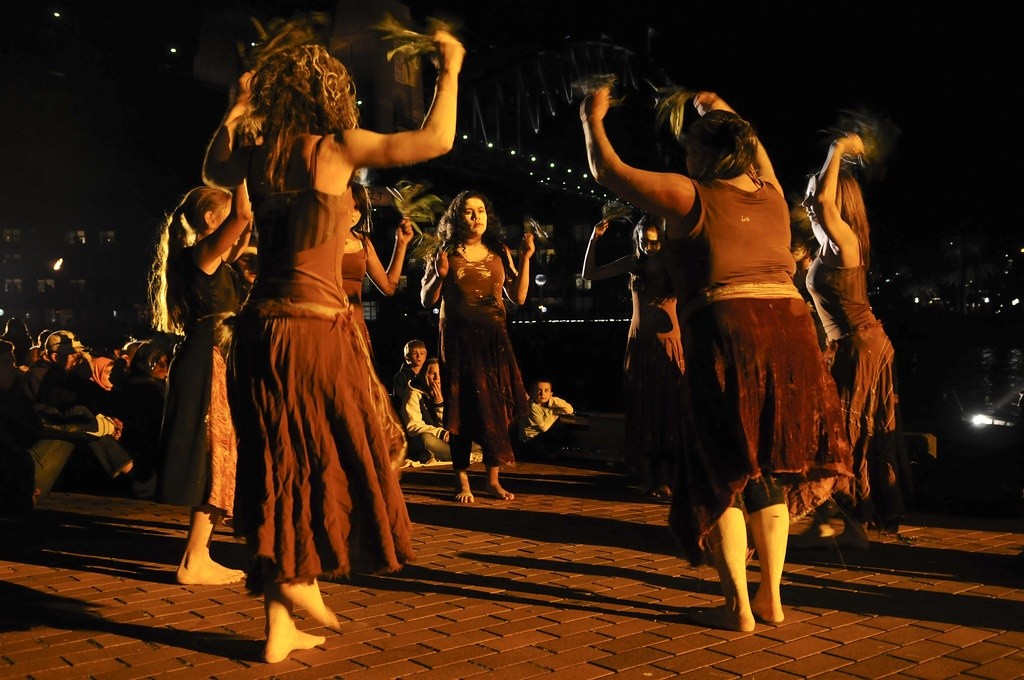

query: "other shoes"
[470,452,484,466]
[642,486,674,501]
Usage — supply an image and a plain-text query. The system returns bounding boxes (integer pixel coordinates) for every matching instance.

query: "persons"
[7,14,898,662]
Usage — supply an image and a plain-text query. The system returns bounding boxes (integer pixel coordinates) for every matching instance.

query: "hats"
[45,330,87,354]
